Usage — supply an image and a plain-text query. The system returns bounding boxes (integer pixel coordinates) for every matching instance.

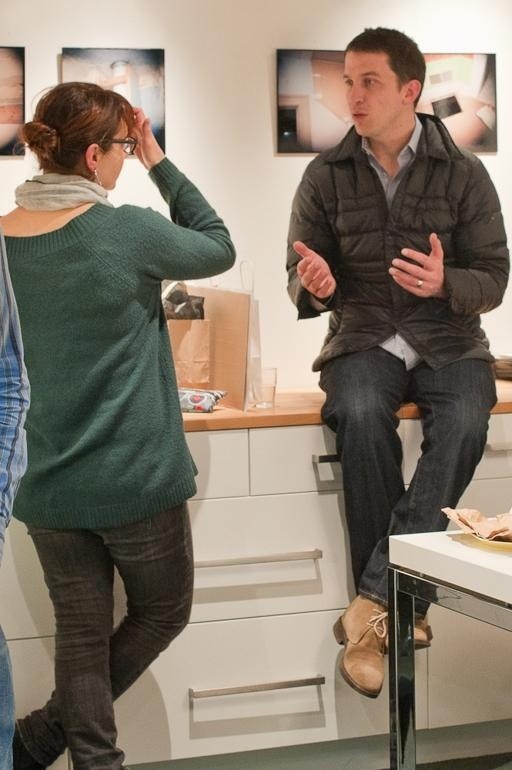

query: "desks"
[382,522,512,770]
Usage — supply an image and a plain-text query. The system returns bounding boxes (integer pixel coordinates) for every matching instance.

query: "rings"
[417,280,424,287]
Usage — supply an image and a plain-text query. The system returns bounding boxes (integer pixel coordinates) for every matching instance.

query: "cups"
[250,366,280,409]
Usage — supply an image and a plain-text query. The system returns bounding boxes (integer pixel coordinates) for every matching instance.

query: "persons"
[0,79,240,770]
[0,227,34,769]
[284,21,507,701]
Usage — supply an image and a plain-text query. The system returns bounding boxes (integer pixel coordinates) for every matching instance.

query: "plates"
[467,515,512,547]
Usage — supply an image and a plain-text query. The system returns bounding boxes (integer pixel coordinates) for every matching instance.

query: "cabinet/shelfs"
[1,373,511,770]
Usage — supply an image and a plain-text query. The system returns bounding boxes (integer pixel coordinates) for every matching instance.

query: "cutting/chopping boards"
[170,283,252,415]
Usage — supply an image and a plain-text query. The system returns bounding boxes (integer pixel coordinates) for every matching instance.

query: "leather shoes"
[332,590,389,700]
[413,612,435,651]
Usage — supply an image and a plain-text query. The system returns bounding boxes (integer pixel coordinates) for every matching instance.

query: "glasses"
[97,136,138,156]
